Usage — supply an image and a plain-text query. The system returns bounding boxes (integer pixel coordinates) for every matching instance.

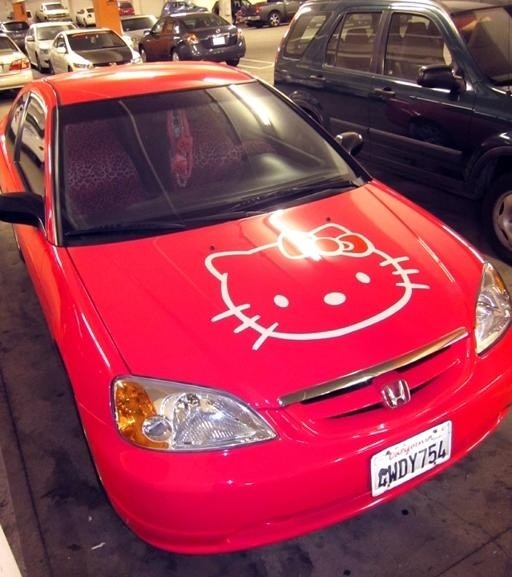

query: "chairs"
[345,21,445,79]
[61,94,250,235]
